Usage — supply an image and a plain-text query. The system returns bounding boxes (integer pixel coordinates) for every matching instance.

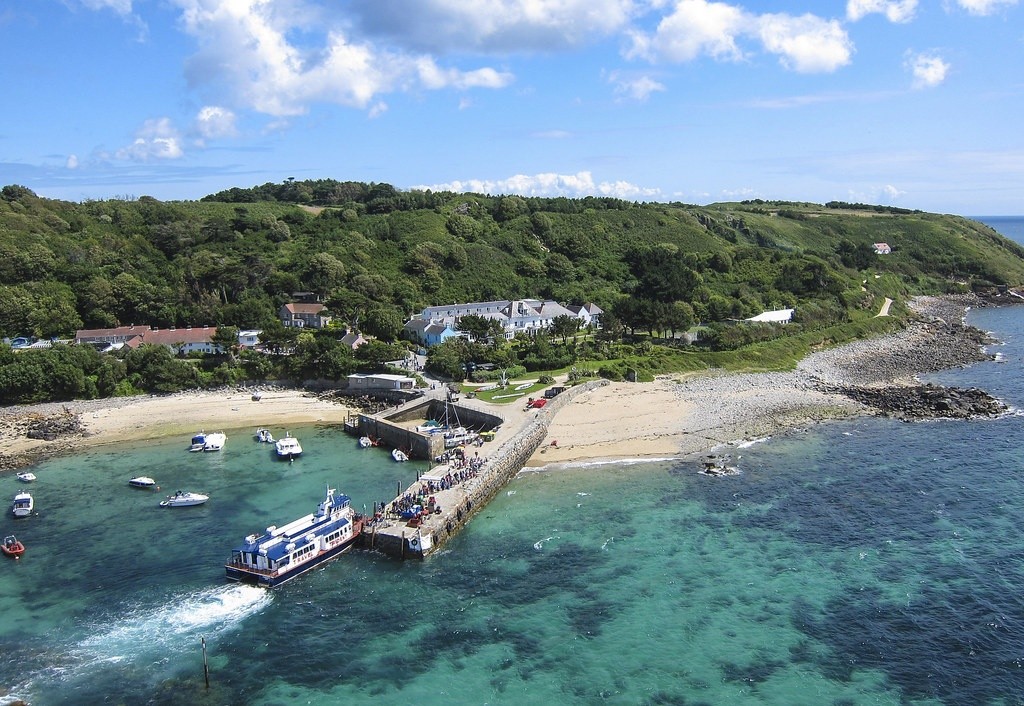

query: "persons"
[378,438,487,520]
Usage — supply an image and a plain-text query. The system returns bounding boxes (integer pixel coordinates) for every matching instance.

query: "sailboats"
[418,384,479,448]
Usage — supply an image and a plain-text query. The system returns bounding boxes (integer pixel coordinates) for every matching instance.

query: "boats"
[226,485,365,588]
[358,435,381,447]
[17,470,37,483]
[159,490,210,509]
[0,535,25,557]
[189,432,204,452]
[205,431,228,453]
[256,426,276,443]
[10,491,34,517]
[273,436,302,456]
[392,447,408,462]
[128,474,155,490]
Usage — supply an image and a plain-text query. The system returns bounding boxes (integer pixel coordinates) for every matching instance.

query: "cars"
[8,338,31,348]
